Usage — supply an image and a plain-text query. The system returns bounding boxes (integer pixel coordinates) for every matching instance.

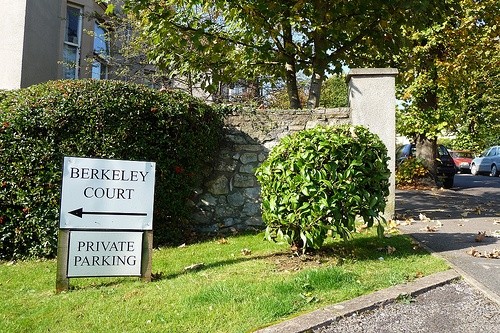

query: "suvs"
[395,143,457,189]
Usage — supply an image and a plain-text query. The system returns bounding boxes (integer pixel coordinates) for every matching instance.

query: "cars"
[471,145,500,177]
[449,150,473,173]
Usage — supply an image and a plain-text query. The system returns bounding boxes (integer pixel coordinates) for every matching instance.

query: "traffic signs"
[60,157,156,230]
